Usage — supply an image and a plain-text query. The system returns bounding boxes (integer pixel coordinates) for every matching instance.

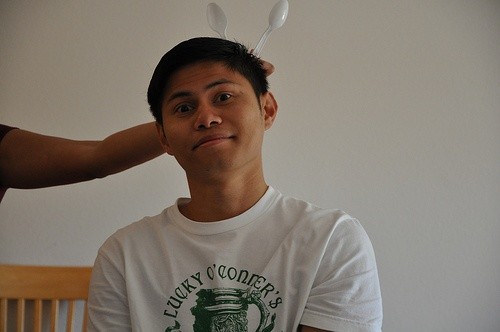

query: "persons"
[0,52,277,202]
[85,37,386,332]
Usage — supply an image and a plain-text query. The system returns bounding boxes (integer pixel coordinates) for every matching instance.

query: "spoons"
[206,2,228,40]
[252,0,289,58]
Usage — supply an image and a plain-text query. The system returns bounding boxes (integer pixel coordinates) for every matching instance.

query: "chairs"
[0,263,93,332]
